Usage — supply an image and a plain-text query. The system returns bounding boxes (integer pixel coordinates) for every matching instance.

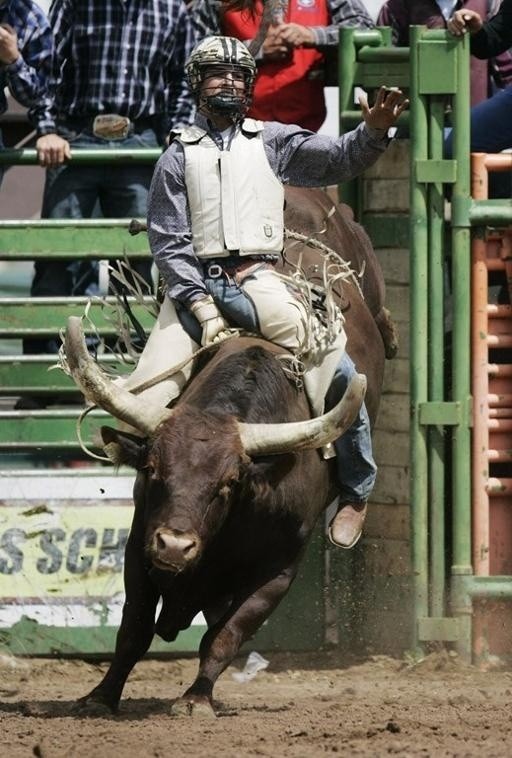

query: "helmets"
[185,36,258,117]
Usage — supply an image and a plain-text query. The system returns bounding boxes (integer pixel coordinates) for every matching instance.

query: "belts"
[206,258,258,278]
[64,110,152,139]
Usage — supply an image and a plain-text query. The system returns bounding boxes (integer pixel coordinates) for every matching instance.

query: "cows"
[55,181,401,722]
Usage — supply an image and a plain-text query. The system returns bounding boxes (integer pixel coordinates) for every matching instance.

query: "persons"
[189,0,373,135]
[1,0,55,184]
[366,0,512,139]
[102,36,407,549]
[443,1,512,206]
[28,0,200,219]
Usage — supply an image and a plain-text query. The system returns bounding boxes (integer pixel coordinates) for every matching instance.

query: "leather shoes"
[329,498,368,548]
